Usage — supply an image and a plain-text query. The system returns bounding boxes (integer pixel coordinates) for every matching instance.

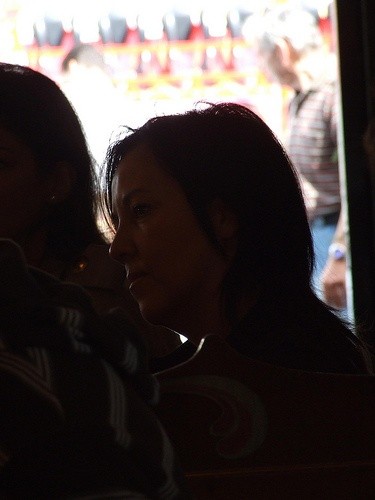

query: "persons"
[0,63,185,358]
[0,234,179,500]
[241,4,356,328]
[59,48,119,242]
[107,99,375,500]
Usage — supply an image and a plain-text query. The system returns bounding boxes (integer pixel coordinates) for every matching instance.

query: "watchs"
[328,240,348,261]
